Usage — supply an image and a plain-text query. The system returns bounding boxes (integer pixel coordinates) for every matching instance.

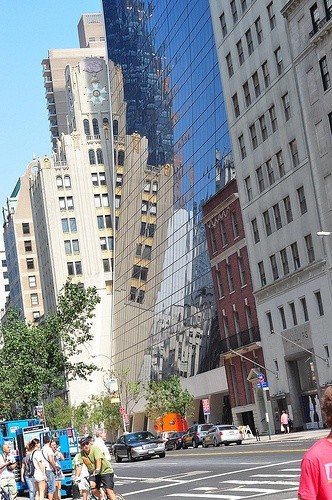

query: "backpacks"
[27,450,36,479]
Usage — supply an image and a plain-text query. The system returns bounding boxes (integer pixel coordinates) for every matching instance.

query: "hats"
[7,462,17,471]
[79,437,89,446]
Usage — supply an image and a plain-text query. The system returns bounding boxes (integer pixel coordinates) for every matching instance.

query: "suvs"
[180,423,213,449]
[157,430,177,446]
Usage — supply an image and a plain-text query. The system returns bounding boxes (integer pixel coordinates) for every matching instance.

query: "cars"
[105,441,113,453]
[165,432,185,451]
[201,425,243,448]
[111,430,166,463]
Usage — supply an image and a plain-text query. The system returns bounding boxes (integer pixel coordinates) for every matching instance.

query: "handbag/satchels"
[281,425,285,431]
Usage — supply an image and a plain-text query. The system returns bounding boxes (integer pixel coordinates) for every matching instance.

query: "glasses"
[83,443,89,447]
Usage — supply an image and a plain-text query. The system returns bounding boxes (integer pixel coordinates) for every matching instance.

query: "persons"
[72,436,118,500]
[281,410,293,434]
[298,386,332,500]
[216,420,220,425]
[21,435,64,500]
[0,443,17,500]
[193,421,198,426]
